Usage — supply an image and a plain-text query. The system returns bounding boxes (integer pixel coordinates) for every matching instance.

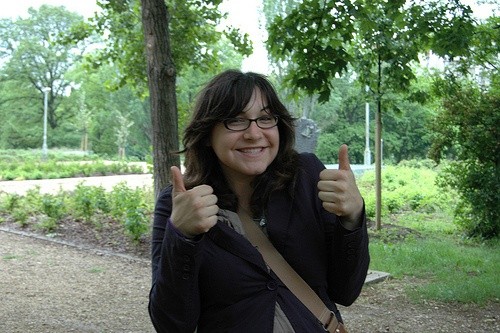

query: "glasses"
[220,114,280,130]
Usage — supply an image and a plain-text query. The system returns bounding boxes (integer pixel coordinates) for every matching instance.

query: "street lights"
[41,87,51,162]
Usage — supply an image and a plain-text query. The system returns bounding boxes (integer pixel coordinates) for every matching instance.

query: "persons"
[147,70,371,333]
